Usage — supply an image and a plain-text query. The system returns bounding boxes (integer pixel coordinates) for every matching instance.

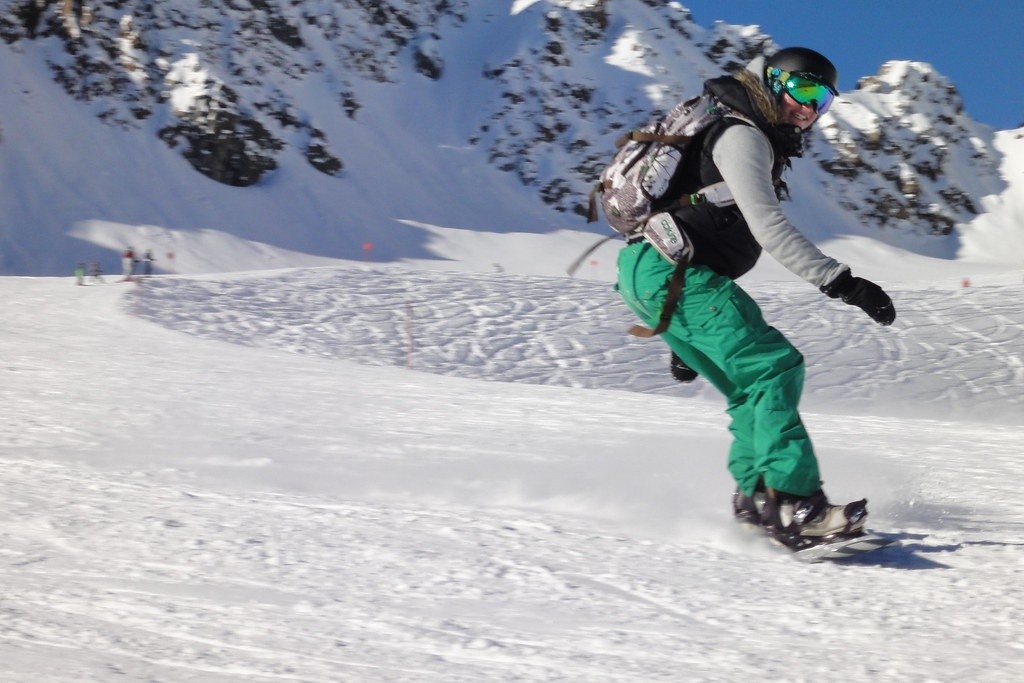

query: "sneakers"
[733,485,868,550]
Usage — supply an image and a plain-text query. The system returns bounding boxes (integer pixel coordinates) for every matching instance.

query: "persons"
[616,46,898,553]
[119,246,154,276]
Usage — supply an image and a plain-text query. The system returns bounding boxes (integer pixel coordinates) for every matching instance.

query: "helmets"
[766,46,841,97]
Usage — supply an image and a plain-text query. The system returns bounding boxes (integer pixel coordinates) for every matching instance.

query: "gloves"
[671,350,700,384]
[821,270,897,326]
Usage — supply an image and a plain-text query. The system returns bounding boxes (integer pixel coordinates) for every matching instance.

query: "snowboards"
[738,503,910,562]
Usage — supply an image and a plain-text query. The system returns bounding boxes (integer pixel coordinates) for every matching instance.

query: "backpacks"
[586,92,731,268]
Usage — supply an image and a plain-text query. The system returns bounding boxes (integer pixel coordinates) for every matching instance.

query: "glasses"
[768,71,836,117]
